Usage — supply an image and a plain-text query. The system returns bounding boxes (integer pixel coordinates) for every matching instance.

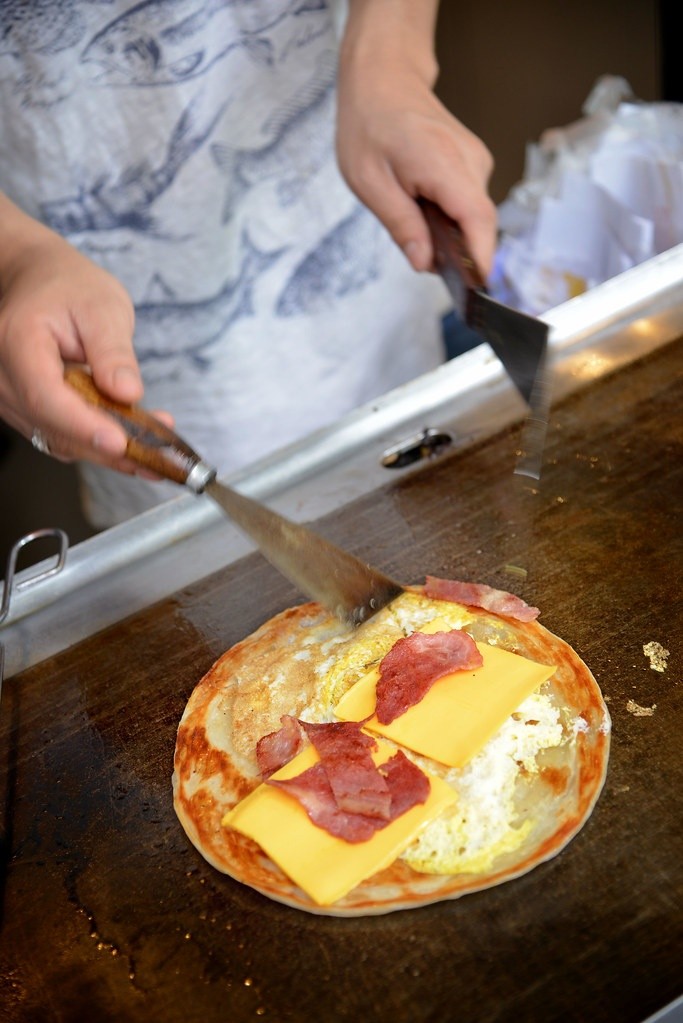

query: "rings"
[32,428,49,454]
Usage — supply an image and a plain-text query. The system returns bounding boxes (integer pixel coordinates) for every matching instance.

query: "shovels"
[60,364,407,631]
[414,192,549,420]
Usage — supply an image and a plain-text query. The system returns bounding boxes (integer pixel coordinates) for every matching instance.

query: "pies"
[171,584,610,916]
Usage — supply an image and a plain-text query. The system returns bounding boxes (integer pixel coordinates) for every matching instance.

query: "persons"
[0,0,500,541]
